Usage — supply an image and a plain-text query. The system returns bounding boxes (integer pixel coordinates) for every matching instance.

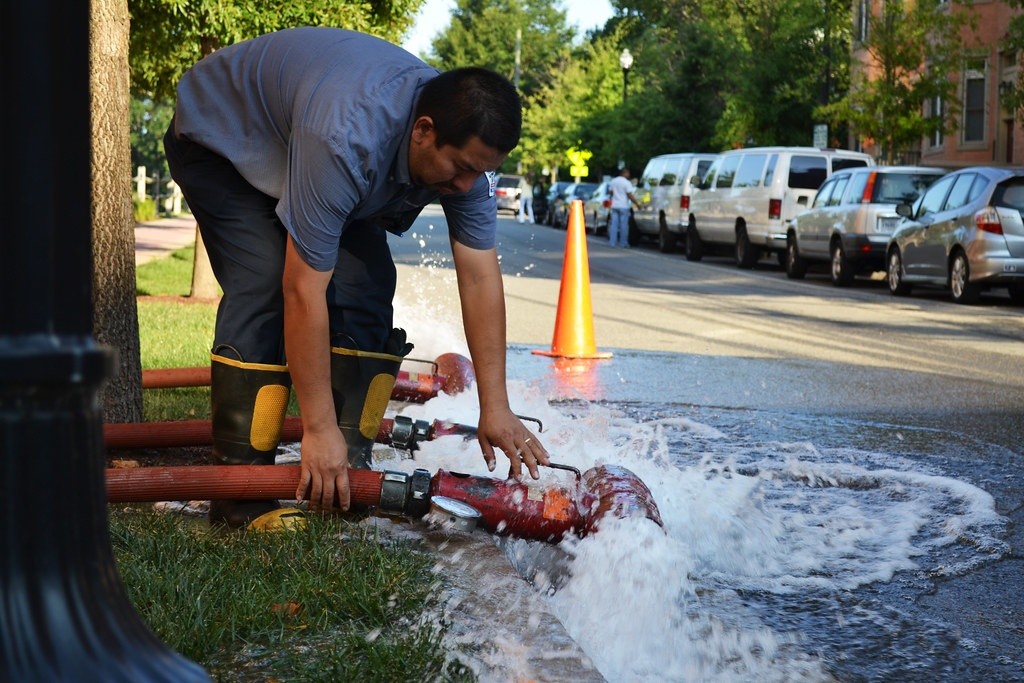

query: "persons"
[516,173,538,225]
[160,27,550,541]
[605,169,641,248]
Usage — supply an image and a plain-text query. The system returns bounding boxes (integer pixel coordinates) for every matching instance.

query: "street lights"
[620,48,633,161]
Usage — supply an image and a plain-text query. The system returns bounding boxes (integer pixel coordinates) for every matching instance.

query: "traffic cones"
[529,198,614,357]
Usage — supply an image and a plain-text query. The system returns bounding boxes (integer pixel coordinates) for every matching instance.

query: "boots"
[329,328,415,472]
[208,345,292,529]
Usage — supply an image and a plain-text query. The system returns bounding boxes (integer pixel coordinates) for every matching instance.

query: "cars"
[494,174,613,237]
[886,165,1023,304]
[786,166,947,286]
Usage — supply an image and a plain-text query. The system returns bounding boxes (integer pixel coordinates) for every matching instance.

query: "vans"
[627,154,721,253]
[685,148,877,270]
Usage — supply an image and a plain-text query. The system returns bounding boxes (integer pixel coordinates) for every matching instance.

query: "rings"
[524,437,532,443]
